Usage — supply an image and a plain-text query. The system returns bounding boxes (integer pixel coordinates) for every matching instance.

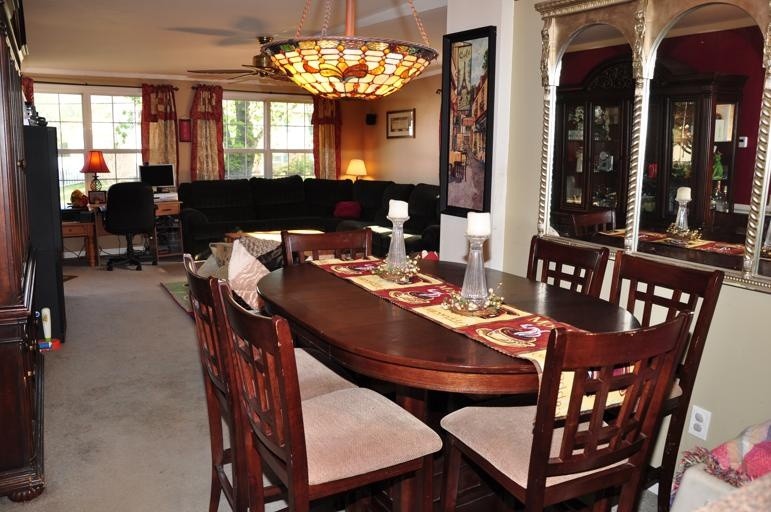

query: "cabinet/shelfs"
[0,1,48,504]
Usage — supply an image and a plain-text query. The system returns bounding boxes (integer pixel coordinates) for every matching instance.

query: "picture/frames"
[437,25,498,219]
[178,117,194,143]
[385,107,416,140]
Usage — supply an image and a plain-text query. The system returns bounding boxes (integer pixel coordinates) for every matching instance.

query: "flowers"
[374,254,424,282]
[449,283,506,310]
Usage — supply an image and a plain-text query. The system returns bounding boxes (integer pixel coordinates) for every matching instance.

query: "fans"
[186,41,293,83]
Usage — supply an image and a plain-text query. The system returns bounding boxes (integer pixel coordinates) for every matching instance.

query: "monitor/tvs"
[139,164,176,193]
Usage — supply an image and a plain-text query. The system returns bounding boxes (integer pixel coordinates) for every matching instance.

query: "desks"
[225,228,325,271]
[61,217,99,269]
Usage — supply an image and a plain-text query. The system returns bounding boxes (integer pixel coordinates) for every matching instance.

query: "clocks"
[88,191,107,204]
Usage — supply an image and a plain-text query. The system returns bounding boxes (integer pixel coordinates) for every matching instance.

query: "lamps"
[264,0,440,100]
[347,159,367,181]
[81,149,108,191]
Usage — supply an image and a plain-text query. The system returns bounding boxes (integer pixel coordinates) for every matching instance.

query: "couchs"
[176,173,442,254]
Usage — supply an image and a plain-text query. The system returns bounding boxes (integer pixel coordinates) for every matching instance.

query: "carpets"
[158,278,198,320]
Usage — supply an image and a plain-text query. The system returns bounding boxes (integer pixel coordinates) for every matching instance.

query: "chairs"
[279,227,373,268]
[181,251,359,512]
[217,282,443,507]
[439,307,694,507]
[525,234,610,298]
[102,179,156,272]
[606,249,726,512]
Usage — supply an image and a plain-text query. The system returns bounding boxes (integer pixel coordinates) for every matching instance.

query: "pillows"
[334,201,372,220]
